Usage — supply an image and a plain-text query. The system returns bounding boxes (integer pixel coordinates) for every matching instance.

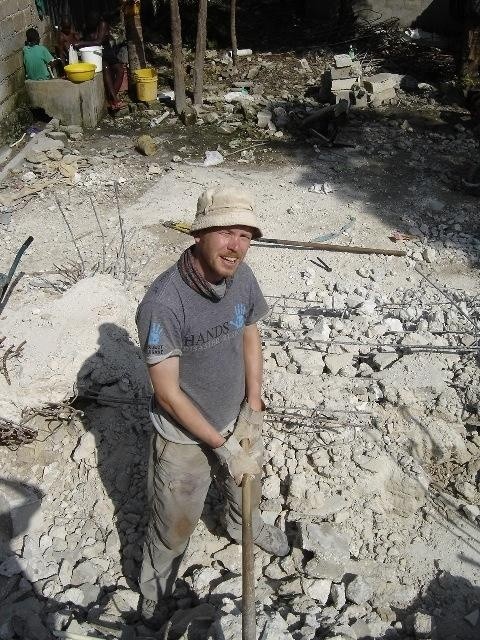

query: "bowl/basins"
[63,62,98,82]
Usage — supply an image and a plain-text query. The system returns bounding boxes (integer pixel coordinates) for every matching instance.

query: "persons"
[23,29,62,82]
[73,9,124,112]
[58,15,83,68]
[135,185,293,631]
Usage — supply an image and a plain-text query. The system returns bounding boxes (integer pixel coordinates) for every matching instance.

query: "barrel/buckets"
[79,45,103,73]
[134,68,158,101]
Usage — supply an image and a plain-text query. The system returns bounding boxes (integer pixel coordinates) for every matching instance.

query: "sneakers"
[140,598,168,630]
[235,523,291,557]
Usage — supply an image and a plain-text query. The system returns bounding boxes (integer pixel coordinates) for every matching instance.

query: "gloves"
[211,429,261,487]
[233,396,266,450]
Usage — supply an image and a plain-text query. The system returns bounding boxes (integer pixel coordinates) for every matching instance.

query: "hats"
[188,186,264,240]
